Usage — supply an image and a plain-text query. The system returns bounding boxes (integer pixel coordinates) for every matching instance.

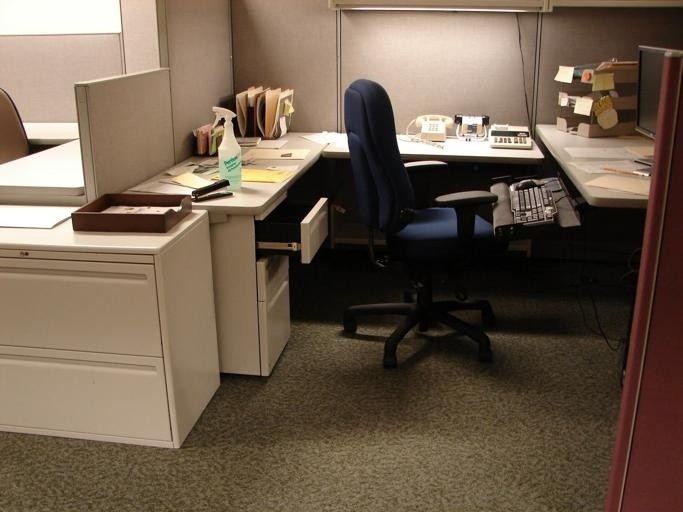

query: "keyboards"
[511,186,558,226]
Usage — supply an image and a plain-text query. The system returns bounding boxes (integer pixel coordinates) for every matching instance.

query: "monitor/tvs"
[634,44,672,177]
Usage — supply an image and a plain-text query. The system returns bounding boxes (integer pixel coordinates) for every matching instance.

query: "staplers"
[192,180,233,201]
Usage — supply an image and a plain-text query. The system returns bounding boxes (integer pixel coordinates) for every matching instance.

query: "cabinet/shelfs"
[1,196,223,453]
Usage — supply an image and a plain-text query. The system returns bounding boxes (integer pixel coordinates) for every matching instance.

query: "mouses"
[516,177,545,190]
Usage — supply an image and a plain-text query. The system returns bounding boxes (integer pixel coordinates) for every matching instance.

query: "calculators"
[489,124,532,149]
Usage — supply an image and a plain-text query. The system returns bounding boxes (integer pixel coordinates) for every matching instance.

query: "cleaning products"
[212,106,242,190]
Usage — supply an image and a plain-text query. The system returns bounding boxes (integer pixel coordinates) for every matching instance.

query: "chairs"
[331,76,511,374]
[0,85,34,166]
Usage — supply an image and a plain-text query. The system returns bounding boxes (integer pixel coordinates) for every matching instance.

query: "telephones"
[416,115,454,142]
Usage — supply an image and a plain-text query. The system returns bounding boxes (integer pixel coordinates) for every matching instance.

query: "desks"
[0,118,86,204]
[535,115,656,218]
[125,119,549,387]
